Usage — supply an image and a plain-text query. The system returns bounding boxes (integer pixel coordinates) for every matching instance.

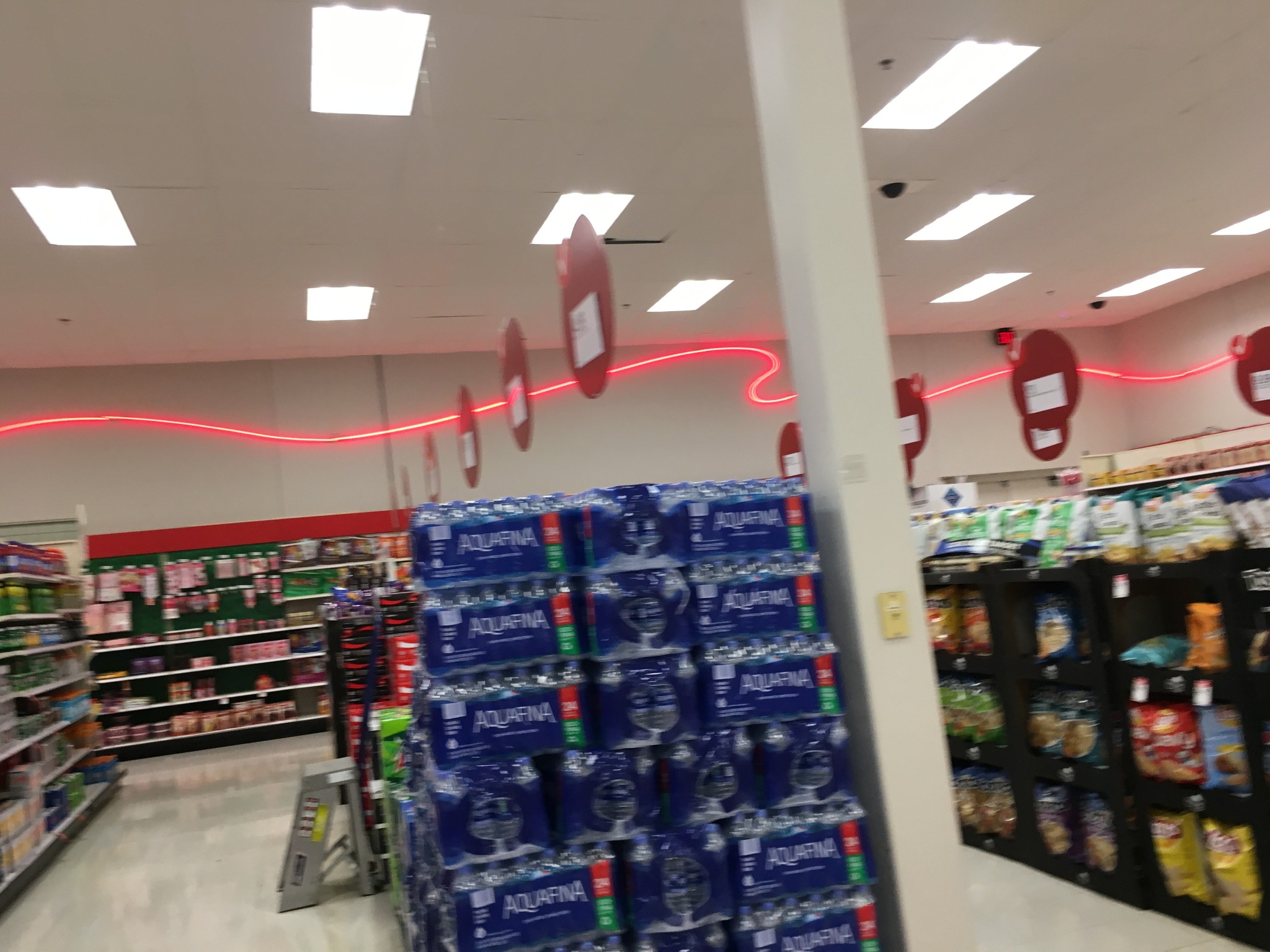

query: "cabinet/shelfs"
[0,554,129,914]
[85,522,410,753]
[912,457,1270,951]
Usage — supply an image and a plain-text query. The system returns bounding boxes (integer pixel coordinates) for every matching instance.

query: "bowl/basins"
[101,721,172,746]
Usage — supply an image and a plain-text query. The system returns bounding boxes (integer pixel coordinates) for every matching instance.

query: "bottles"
[407,479,884,952]
[0,540,80,654]
